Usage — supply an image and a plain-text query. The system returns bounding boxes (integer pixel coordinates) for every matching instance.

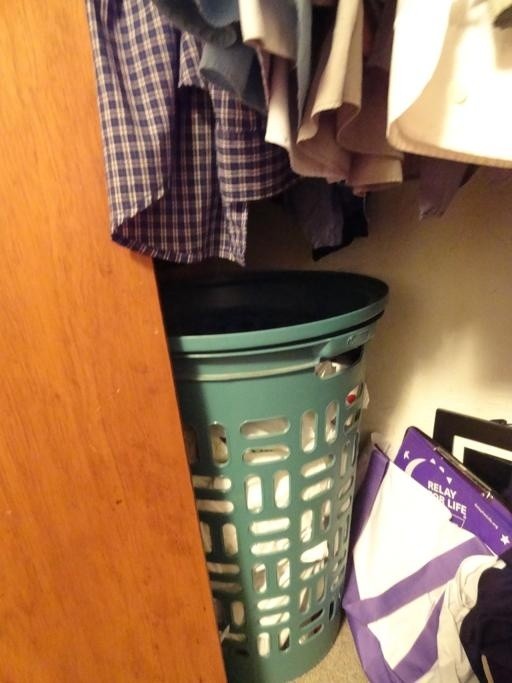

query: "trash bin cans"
[156,269,389,683]
